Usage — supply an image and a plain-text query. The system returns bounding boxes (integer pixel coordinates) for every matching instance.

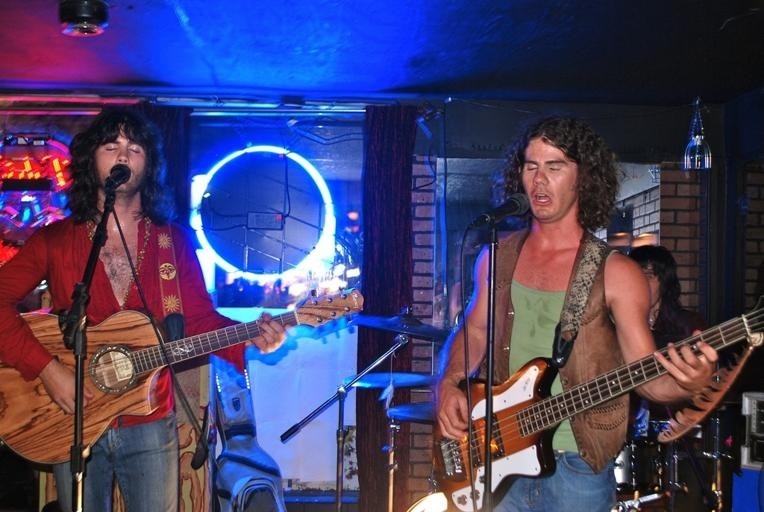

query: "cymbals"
[387,400,438,425]
[343,370,433,390]
[352,304,452,343]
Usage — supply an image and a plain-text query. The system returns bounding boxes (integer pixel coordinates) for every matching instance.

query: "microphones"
[472,193,529,227]
[104,164,131,195]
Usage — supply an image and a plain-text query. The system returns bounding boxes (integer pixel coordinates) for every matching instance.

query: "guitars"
[0,286,366,466]
[427,299,764,512]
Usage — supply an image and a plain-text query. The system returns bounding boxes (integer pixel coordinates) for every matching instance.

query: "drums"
[614,438,684,492]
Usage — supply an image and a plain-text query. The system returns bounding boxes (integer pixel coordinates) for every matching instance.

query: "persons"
[431,113,717,511]
[624,243,714,511]
[0,105,290,511]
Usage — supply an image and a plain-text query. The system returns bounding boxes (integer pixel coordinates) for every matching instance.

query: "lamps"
[683,95,712,170]
